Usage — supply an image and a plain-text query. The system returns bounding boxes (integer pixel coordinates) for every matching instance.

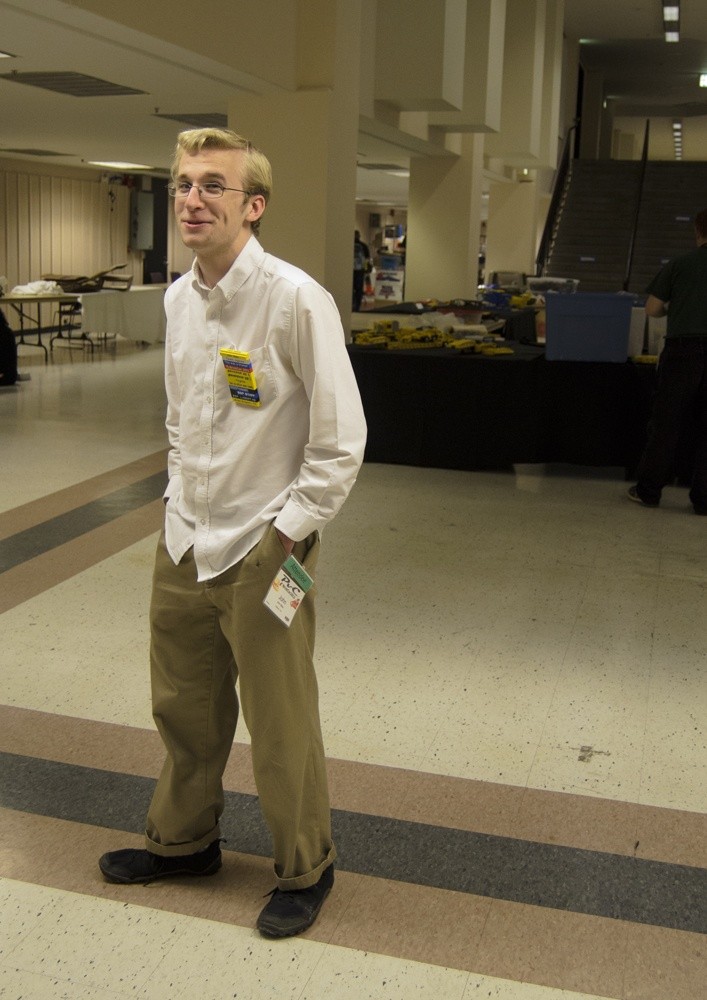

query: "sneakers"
[256,863,335,937]
[98,837,227,883]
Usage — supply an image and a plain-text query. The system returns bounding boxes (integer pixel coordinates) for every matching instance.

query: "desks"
[0,281,174,365]
[347,341,688,484]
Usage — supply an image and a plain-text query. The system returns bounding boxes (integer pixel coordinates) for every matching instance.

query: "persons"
[629,218,707,513]
[94,133,368,939]
[351,226,407,313]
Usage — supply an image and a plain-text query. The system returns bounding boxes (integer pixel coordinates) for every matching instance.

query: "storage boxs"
[542,284,637,365]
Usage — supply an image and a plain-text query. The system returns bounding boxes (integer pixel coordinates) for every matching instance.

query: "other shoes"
[627,486,660,506]
[695,504,707,514]
[0,372,17,386]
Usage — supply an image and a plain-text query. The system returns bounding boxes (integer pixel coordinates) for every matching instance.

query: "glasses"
[166,181,252,199]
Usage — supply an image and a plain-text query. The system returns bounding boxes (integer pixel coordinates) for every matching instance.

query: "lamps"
[661,0,681,44]
[672,119,683,160]
